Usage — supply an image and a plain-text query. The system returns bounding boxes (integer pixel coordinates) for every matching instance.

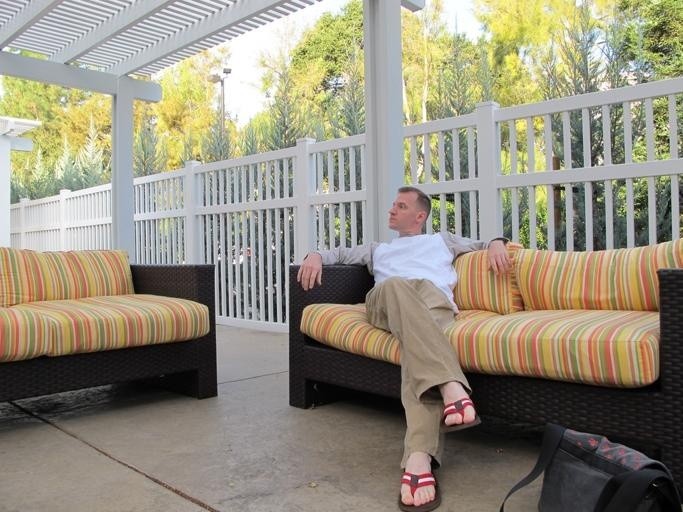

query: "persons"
[296,186,512,511]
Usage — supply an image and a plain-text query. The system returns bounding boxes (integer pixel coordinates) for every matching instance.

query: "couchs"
[0,242,222,403]
[283,235,683,512]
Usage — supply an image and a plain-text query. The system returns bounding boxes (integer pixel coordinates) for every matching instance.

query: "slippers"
[398,468,441,512]
[441,399,480,432]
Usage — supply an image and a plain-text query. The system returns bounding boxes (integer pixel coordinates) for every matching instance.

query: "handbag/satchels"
[538,422,680,511]
